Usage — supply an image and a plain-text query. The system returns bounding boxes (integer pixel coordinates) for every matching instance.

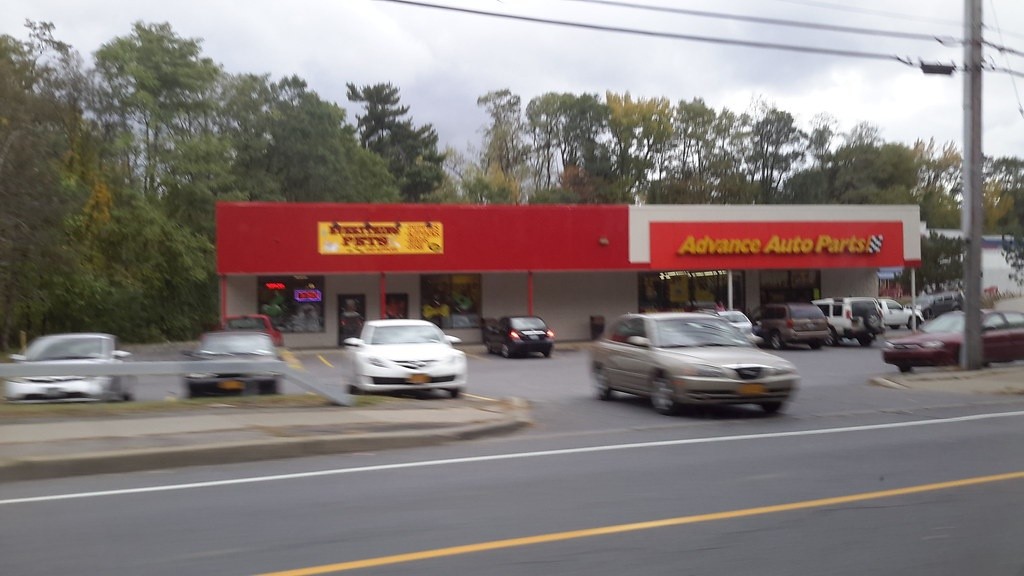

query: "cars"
[878,292,964,329]
[884,310,1024,373]
[588,312,801,415]
[5,332,134,403]
[180,314,286,396]
[484,316,555,358]
[715,311,763,346]
[343,319,469,398]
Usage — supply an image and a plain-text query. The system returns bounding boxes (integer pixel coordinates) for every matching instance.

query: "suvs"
[751,299,830,350]
[812,297,924,346]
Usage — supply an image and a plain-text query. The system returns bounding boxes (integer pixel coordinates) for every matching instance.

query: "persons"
[340,299,363,344]
[715,298,726,314]
